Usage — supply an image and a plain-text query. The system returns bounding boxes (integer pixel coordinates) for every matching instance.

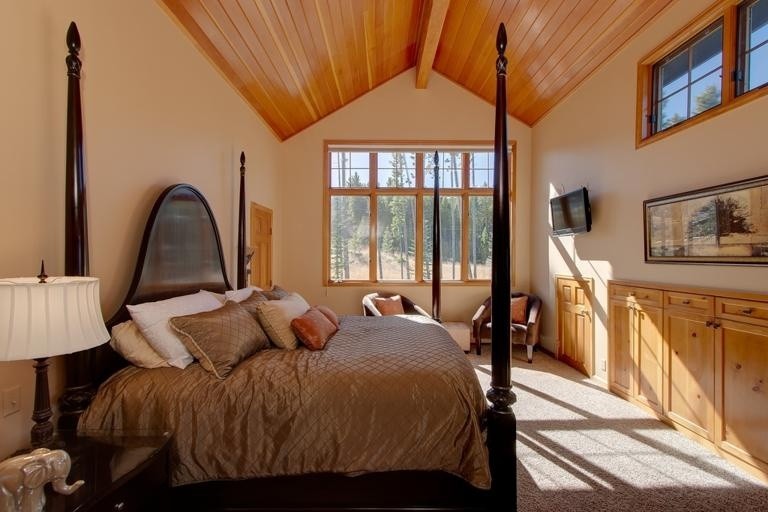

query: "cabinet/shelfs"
[553,275,593,379]
[663,286,764,485]
[606,278,663,421]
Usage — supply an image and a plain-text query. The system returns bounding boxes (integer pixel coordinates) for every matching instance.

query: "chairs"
[471,290,546,367]
[361,288,438,330]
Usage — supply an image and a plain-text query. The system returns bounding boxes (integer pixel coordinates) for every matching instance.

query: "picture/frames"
[641,172,768,271]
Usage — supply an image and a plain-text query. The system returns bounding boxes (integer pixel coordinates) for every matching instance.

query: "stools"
[440,319,475,355]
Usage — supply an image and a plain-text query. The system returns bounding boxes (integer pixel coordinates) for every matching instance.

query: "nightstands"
[0,423,183,511]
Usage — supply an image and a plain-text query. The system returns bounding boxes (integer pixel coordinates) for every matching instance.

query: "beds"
[61,17,521,512]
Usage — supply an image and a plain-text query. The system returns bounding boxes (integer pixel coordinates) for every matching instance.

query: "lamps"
[1,256,114,454]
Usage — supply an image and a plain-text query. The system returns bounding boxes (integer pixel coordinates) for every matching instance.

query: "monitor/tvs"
[550,187,592,236]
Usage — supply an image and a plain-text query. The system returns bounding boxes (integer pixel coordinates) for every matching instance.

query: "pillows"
[106,283,344,385]
[373,293,407,317]
[509,294,529,326]
